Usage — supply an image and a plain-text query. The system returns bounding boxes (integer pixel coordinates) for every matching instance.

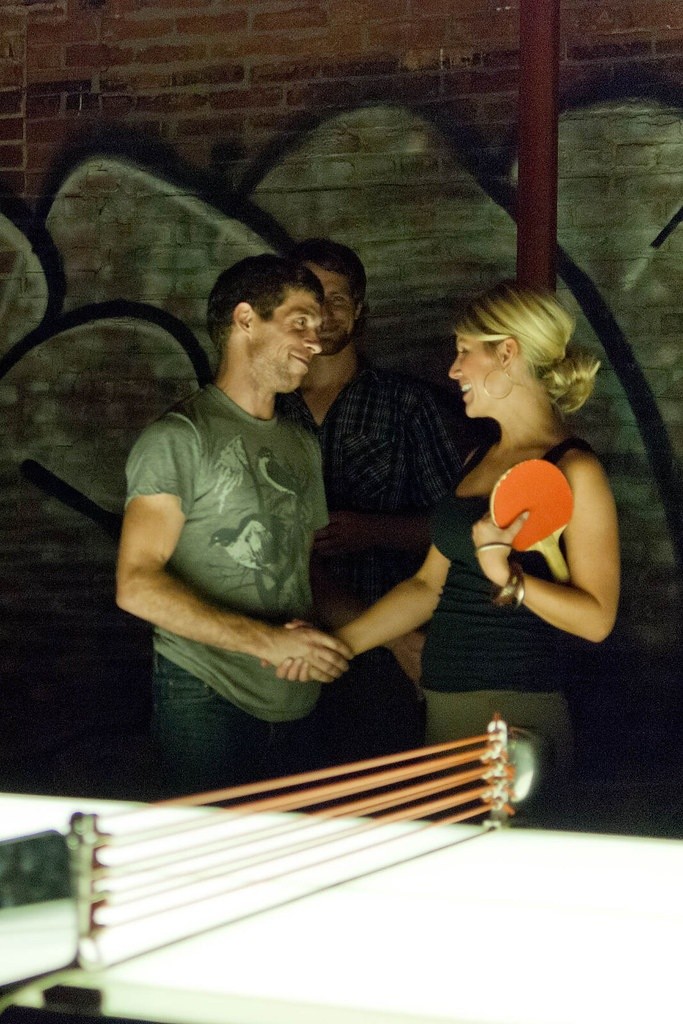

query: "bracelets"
[475,542,512,557]
[512,562,525,609]
[492,570,520,606]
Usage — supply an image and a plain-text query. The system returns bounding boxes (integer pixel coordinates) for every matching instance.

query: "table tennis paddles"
[490,459,574,585]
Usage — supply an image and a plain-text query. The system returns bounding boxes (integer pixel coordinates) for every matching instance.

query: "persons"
[273,238,472,795]
[113,252,354,815]
[280,278,621,826]
